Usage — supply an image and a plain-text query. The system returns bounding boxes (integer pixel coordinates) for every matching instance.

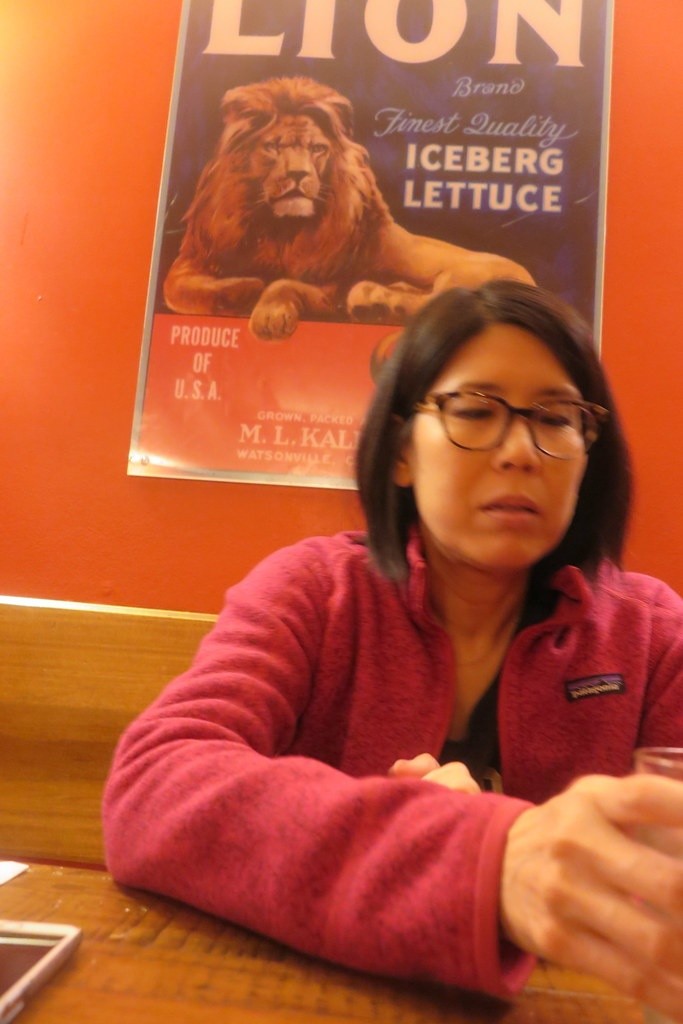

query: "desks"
[0,862,650,1024]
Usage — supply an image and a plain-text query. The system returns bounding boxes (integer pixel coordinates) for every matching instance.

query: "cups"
[630,747,683,857]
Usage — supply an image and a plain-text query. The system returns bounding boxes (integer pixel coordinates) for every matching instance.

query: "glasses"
[412,392,611,460]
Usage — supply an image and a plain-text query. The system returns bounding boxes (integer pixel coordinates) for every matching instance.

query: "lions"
[162,76,538,340]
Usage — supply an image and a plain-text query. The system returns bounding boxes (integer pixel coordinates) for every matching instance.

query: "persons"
[101,280,683,1024]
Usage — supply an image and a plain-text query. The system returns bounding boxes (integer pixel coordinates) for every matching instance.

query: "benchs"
[1,595,220,862]
